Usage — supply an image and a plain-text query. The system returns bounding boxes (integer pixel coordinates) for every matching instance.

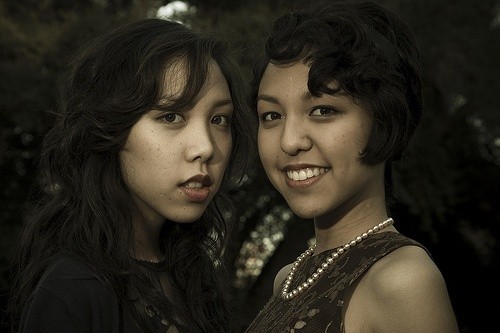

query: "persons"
[13,19,250,333]
[244,0,460,333]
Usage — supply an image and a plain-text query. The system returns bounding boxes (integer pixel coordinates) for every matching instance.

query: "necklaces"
[280,216,395,300]
